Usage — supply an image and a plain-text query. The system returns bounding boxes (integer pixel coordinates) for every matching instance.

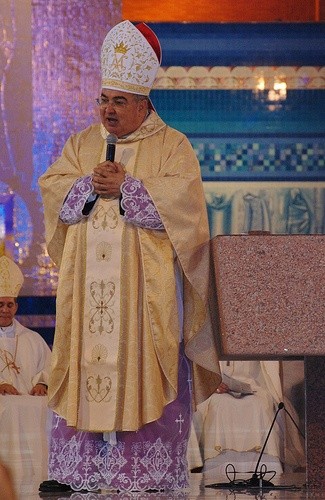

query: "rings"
[217,389,220,392]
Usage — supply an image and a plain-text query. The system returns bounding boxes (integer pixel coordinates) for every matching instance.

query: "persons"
[38,20,203,495]
[0,256,59,498]
[214,360,284,476]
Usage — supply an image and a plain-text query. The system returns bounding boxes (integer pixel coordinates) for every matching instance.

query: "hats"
[0,255,24,298]
[99,19,161,96]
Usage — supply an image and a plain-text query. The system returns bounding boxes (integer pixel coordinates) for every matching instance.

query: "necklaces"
[0,320,21,376]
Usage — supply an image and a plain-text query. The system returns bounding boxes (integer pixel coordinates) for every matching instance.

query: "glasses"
[95,95,137,108]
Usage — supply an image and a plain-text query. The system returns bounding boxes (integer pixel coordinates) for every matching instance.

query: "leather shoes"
[39,480,99,494]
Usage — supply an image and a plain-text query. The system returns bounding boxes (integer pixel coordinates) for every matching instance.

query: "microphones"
[106,134,118,162]
[248,402,286,487]
